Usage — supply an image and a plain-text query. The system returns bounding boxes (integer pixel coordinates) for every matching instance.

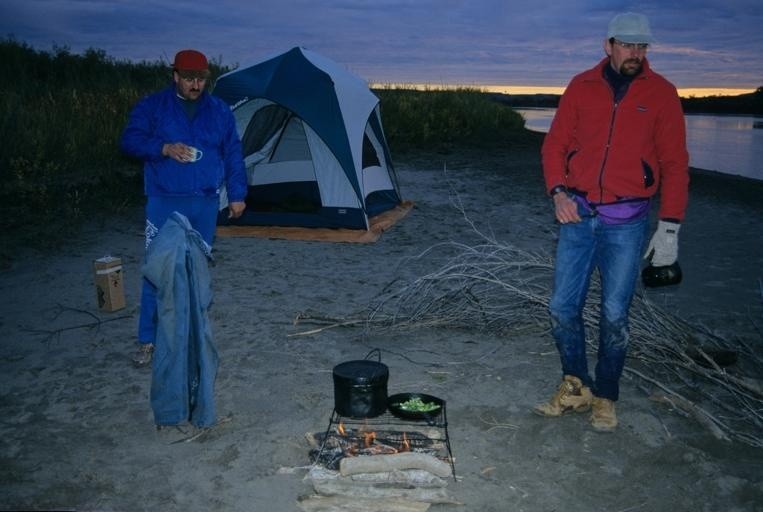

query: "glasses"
[613,41,649,50]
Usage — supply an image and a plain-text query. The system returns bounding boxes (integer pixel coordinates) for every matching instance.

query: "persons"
[531,12,692,431]
[118,48,250,368]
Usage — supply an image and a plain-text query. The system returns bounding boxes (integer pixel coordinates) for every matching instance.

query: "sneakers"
[533,373,594,419]
[132,341,154,368]
[589,395,619,434]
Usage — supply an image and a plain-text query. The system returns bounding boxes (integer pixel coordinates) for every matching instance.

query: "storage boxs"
[95,257,126,312]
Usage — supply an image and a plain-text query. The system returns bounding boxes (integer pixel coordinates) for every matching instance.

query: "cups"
[184,146,203,163]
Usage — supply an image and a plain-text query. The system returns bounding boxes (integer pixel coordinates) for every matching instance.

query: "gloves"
[642,217,683,269]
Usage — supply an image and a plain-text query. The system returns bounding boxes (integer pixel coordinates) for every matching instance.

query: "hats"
[607,11,653,46]
[169,49,209,73]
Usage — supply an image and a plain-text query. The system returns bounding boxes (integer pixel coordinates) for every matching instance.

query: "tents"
[209,44,403,232]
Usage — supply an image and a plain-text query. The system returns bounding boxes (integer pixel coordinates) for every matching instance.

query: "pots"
[387,392,444,428]
[330,348,389,420]
